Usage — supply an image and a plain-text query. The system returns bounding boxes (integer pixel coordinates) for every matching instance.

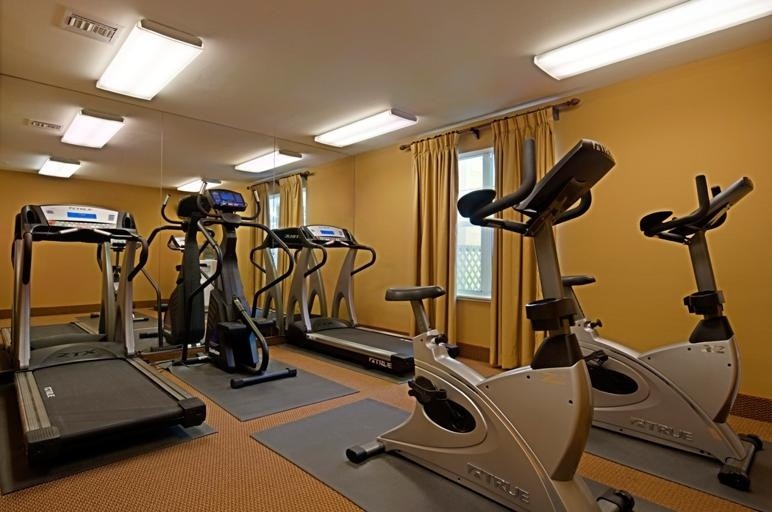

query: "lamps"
[232,149,302,175]
[311,108,418,148]
[92,18,203,102]
[38,154,82,179]
[59,109,126,152]
[176,178,222,194]
[532,1,771,81]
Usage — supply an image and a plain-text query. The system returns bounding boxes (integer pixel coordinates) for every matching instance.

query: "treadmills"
[0,203,207,463]
[244,227,328,334]
[2,209,118,350]
[288,226,458,378]
